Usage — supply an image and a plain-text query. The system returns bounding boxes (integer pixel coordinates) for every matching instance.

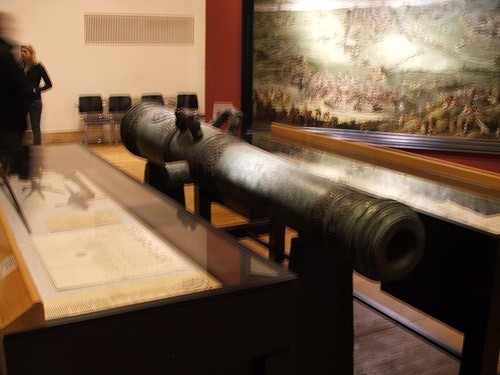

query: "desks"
[0,143,302,375]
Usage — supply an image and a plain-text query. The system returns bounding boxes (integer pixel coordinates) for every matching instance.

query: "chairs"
[78,92,204,147]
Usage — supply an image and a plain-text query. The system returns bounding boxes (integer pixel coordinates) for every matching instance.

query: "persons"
[0,9,27,145]
[18,44,52,145]
